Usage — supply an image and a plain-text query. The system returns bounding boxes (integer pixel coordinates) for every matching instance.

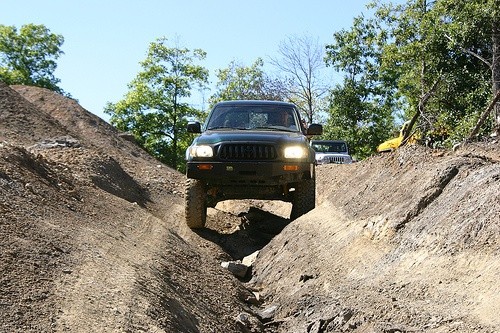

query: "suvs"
[375,118,434,154]
[183,99,324,230]
[308,139,352,165]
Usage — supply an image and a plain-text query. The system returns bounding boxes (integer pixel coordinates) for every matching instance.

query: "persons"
[268,112,306,130]
